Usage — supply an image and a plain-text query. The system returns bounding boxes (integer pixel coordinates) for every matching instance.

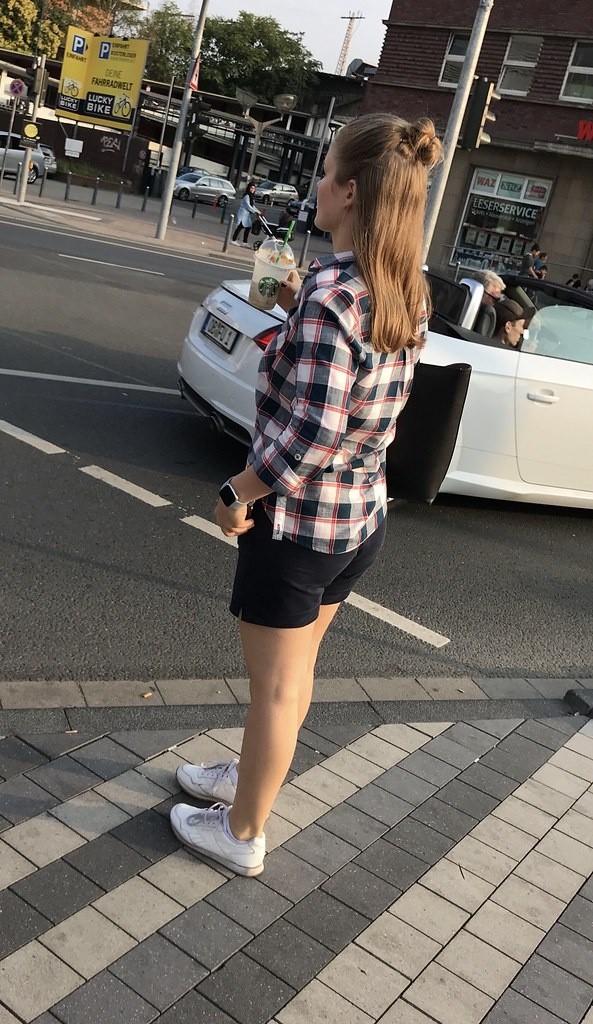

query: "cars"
[173,173,237,208]
[0,131,57,184]
[254,181,299,206]
[176,259,593,509]
[176,166,210,177]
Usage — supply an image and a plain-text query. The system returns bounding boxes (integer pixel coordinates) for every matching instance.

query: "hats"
[494,299,535,328]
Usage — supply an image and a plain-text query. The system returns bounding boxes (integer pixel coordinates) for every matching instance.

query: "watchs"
[219,477,255,509]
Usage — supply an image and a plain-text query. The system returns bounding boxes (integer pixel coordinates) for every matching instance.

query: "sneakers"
[176,758,239,807]
[170,802,266,876]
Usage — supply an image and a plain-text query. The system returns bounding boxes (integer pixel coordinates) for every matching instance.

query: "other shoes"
[242,243,251,247]
[231,241,240,246]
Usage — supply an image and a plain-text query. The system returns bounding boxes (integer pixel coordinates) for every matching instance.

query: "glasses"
[484,291,500,302]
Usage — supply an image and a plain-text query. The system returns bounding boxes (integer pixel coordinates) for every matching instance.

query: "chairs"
[472,303,496,337]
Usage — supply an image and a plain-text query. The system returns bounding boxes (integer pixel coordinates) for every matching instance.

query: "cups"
[249,239,296,310]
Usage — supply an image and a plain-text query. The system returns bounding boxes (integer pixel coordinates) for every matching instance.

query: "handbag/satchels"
[251,220,261,235]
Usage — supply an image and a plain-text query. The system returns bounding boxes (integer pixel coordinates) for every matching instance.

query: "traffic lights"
[461,78,502,151]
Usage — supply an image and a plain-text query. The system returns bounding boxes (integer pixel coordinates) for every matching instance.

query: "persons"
[495,300,536,347]
[230,182,262,249]
[170,113,442,876]
[520,244,593,294]
[469,270,506,305]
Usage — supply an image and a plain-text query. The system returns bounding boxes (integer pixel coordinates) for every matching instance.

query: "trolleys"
[252,213,294,251]
